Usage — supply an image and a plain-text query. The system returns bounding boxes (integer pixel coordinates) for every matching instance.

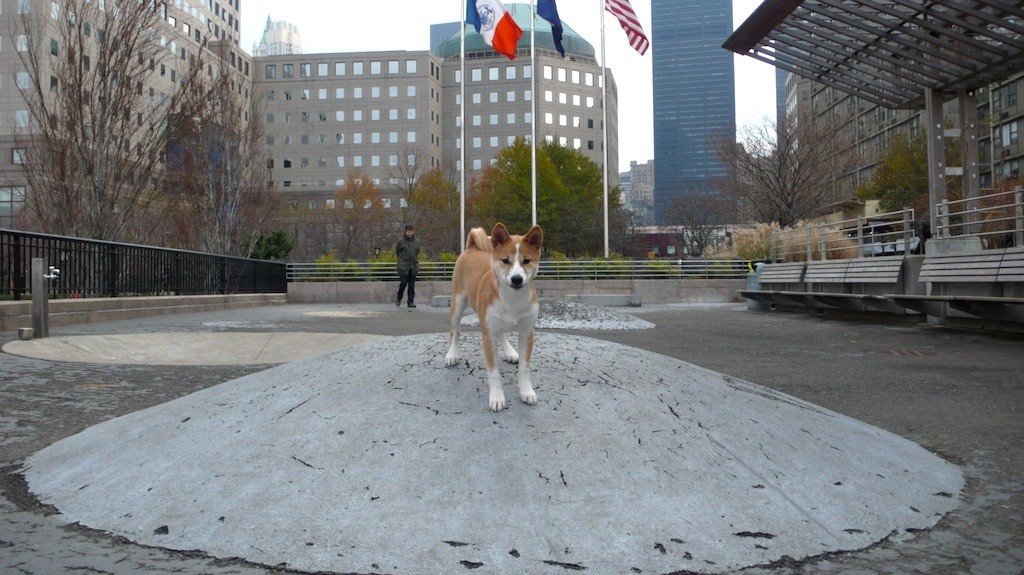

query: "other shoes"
[396,298,401,306]
[408,304,417,308]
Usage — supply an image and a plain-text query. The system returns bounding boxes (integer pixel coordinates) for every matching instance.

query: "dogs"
[444,223,544,412]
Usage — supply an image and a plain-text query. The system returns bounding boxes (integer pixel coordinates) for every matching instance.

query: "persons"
[396,225,420,307]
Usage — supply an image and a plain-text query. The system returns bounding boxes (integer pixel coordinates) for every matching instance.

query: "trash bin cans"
[746,262,767,310]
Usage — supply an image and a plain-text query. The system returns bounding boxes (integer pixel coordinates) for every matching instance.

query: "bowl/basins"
[18,328,34,340]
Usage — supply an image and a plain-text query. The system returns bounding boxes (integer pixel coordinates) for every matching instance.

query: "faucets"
[44,266,61,279]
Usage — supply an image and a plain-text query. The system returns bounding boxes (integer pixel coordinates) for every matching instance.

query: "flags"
[605,0,649,56]
[537,0,566,58]
[466,0,523,60]
